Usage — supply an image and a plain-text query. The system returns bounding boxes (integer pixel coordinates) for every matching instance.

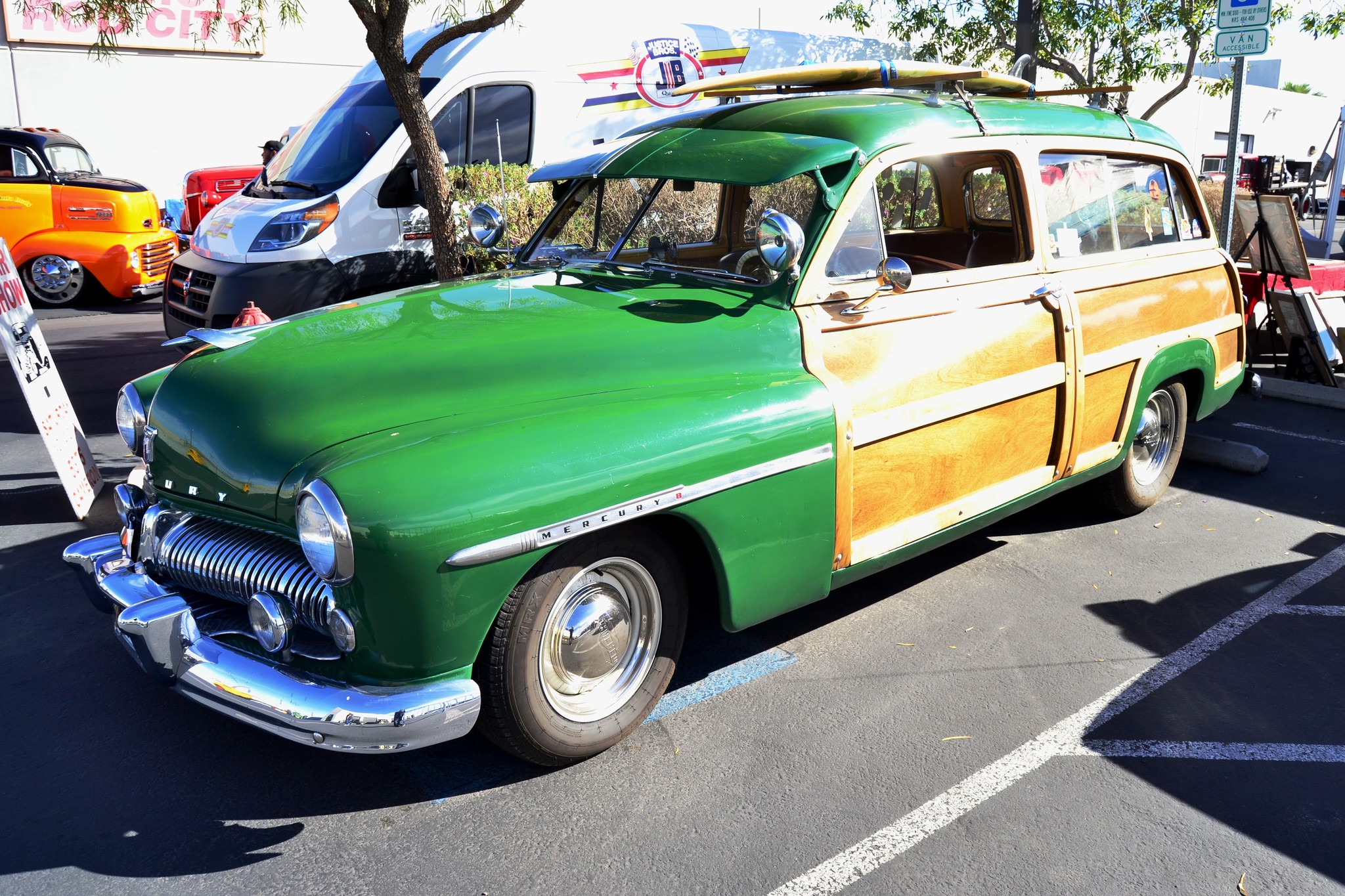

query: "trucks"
[1193,151,1327,221]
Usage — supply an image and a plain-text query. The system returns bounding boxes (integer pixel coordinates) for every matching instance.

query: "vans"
[162,27,937,360]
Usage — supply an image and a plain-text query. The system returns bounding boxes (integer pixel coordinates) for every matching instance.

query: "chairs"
[965,230,1016,270]
[884,232,974,277]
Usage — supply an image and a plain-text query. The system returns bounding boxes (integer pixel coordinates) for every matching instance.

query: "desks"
[1235,253,1345,304]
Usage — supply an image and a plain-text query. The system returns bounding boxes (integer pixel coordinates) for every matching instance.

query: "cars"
[177,125,305,253]
[0,125,182,308]
[60,60,1247,765]
